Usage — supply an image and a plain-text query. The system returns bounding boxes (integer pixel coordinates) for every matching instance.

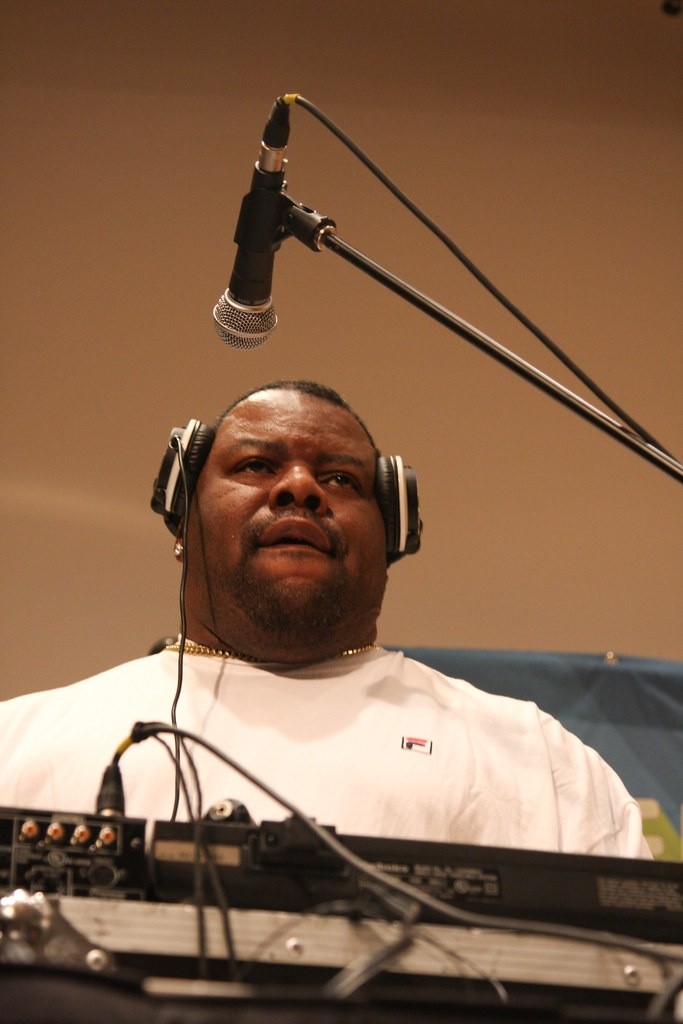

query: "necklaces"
[162,642,376,663]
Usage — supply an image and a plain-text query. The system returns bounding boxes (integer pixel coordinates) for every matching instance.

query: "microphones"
[212,94,291,352]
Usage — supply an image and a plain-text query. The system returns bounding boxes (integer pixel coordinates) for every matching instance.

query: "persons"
[0,380,656,861]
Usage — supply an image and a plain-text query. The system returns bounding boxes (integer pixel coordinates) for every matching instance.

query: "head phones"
[150,419,423,568]
